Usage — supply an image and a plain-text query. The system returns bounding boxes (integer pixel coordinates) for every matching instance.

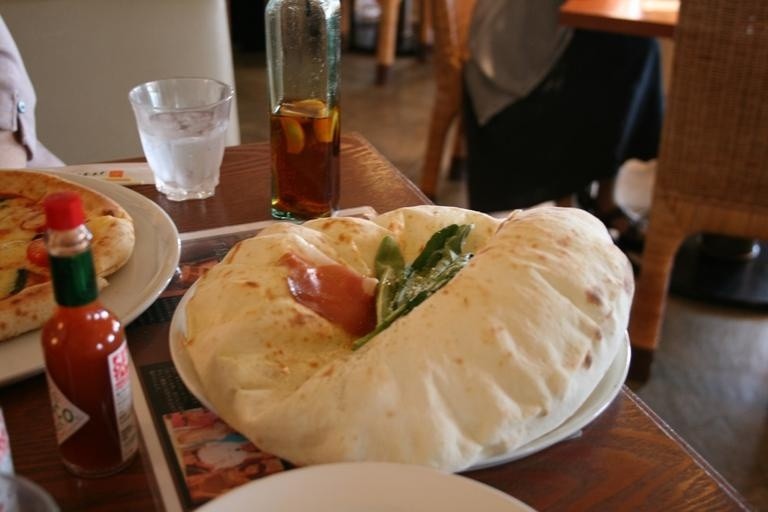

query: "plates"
[0,161,185,386]
[185,458,537,512]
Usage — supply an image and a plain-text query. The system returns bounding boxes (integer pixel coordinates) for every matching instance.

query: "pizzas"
[2,167,135,344]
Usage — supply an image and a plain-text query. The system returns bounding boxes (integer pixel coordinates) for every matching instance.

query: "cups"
[125,75,233,204]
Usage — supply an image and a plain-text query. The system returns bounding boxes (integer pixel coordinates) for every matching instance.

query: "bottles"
[33,192,142,481]
[261,1,345,225]
